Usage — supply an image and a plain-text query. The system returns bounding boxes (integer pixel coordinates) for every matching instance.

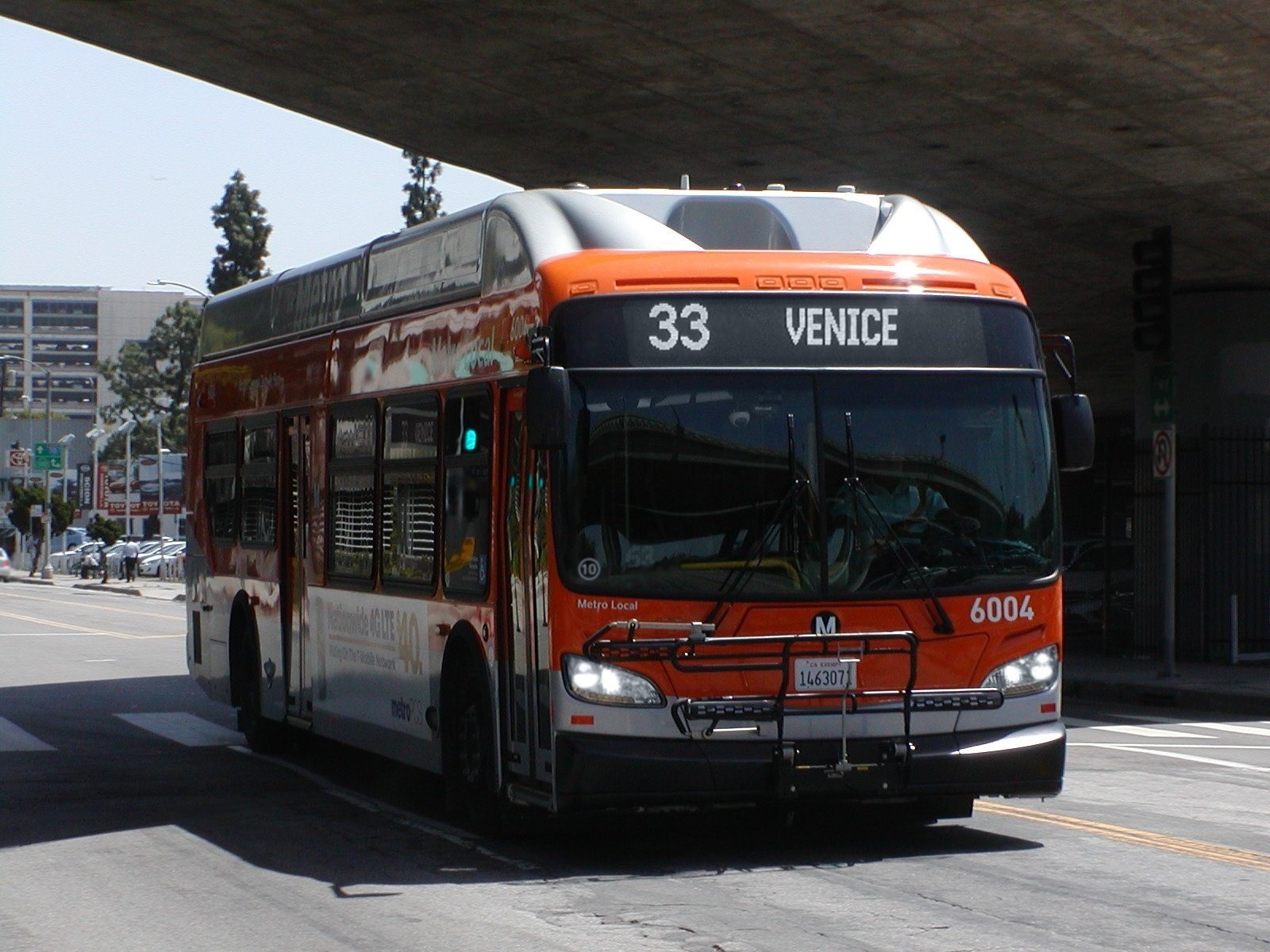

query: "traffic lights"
[1131,224,1172,368]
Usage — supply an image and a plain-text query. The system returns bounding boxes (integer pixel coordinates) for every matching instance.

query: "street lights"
[58,433,76,573]
[19,394,34,552]
[84,427,105,578]
[150,410,173,583]
[0,354,54,579]
[117,419,138,543]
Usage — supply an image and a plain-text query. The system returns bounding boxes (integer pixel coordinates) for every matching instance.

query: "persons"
[123,536,139,582]
[465,493,491,556]
[846,433,950,555]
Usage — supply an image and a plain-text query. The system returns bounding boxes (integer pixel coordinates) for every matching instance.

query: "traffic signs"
[32,441,64,471]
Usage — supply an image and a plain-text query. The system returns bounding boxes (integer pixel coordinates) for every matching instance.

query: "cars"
[25,525,187,583]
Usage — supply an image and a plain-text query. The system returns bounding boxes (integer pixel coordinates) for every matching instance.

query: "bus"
[178,173,1101,842]
